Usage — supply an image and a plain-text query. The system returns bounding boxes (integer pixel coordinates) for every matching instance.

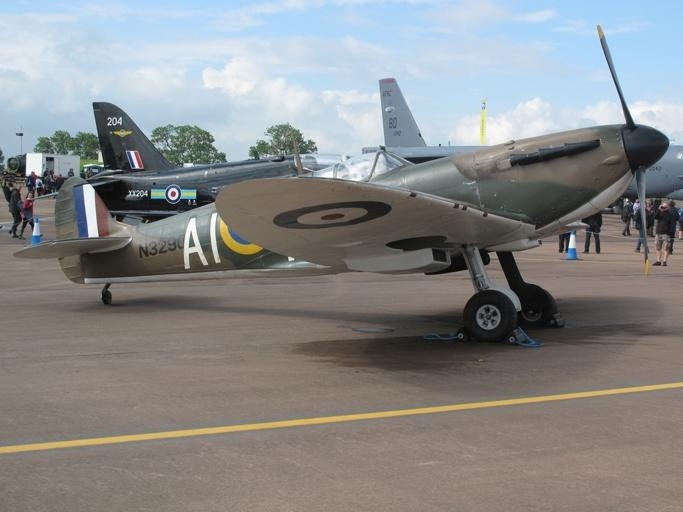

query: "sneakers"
[9,231,25,239]
[663,261,667,266]
[653,262,661,265]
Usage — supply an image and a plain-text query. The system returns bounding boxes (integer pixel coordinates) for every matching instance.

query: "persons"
[557,232,570,253]
[650,201,676,267]
[8,182,24,238]
[617,196,682,255]
[24,166,94,200]
[0,170,14,203]
[580,211,602,254]
[17,190,44,239]
